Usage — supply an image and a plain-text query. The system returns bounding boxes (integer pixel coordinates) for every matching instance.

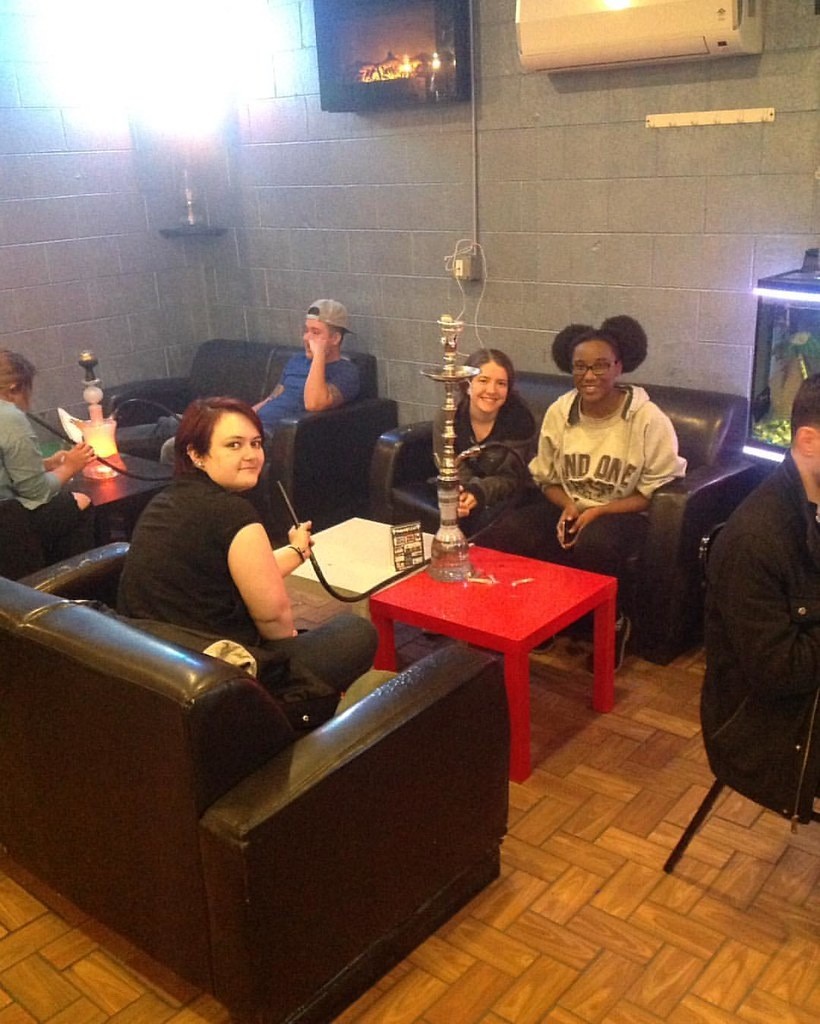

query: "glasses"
[573,360,616,375]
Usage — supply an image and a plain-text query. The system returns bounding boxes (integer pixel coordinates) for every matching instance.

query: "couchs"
[0,542,511,1024]
[370,371,758,668]
[99,339,398,548]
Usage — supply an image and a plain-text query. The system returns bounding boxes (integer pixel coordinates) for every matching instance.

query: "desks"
[272,518,474,621]
[64,452,178,549]
[370,545,619,785]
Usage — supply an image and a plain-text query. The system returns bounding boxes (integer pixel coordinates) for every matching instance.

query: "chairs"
[663,522,820,876]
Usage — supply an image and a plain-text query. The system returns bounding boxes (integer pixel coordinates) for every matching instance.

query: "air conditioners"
[514,0,764,74]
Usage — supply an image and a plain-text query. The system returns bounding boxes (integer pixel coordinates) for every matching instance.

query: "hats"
[306,299,354,335]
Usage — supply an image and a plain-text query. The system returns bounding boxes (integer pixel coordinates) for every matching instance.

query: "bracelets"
[289,545,305,562]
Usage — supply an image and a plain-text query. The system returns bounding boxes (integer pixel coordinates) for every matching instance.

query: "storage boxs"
[743,271,820,462]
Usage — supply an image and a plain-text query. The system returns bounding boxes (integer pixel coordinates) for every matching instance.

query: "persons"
[426,348,538,555]
[528,314,689,676]
[697,372,820,826]
[0,350,117,608]
[161,298,360,478]
[116,394,379,731]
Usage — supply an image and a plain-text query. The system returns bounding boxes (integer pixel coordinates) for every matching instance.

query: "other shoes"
[587,612,631,676]
[533,635,557,654]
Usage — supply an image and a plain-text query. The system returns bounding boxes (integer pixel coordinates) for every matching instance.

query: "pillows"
[116,613,276,687]
